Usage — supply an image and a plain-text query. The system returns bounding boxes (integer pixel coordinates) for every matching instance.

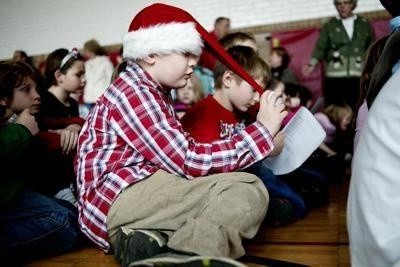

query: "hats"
[122,3,204,60]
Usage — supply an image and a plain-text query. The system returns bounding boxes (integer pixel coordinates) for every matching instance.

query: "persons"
[1,39,127,267]
[301,0,400,267]
[74,3,288,267]
[171,17,353,229]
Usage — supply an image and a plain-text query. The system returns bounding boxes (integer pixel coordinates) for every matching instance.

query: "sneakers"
[113,226,247,267]
